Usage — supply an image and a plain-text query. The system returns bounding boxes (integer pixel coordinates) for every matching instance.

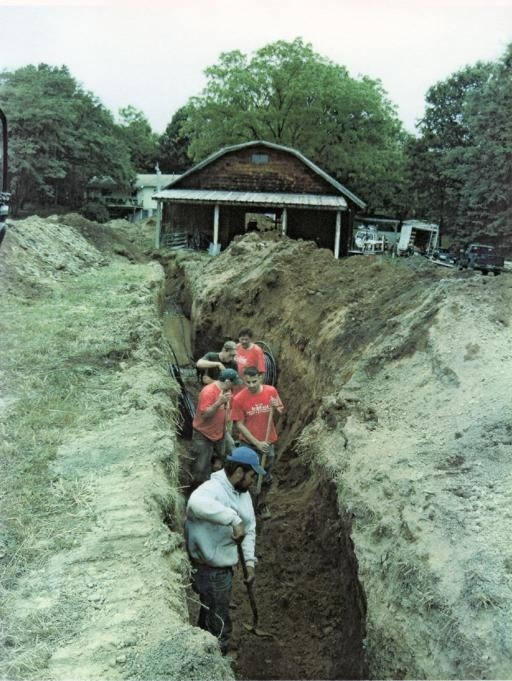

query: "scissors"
[234,539,272,637]
[252,406,275,511]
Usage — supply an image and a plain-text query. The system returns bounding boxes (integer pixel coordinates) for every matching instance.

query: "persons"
[197,340,240,464]
[231,366,284,520]
[231,327,266,444]
[189,367,243,489]
[390,237,401,257]
[185,446,266,661]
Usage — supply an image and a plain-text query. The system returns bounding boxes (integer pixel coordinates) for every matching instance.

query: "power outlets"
[460,244,505,275]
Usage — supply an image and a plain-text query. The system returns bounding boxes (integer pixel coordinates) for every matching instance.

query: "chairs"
[258,502,271,519]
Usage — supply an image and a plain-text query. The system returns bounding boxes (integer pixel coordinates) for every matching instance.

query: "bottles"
[223,341,237,356]
[220,367,242,384]
[226,447,267,476]
